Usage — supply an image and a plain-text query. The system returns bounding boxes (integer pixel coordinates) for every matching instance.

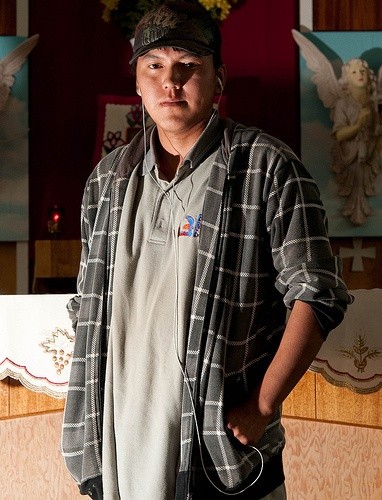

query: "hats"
[129,0,222,65]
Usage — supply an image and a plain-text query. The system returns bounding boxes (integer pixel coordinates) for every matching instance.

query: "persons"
[290,29,382,224]
[60,0,354,500]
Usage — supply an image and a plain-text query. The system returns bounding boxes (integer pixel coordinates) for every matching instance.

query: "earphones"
[216,75,224,91]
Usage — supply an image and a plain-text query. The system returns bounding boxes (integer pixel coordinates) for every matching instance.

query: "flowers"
[101,0,119,23]
[198,0,232,23]
